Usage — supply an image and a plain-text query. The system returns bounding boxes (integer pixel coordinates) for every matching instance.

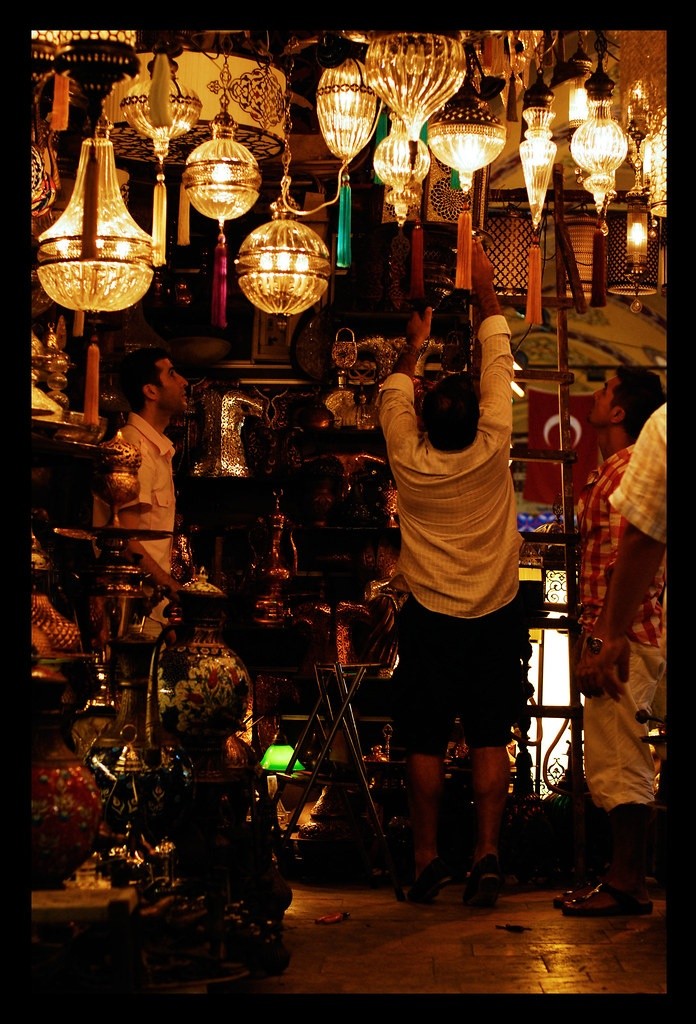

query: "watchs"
[584,633,605,656]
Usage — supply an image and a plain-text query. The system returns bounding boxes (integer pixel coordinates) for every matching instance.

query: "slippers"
[553,881,653,916]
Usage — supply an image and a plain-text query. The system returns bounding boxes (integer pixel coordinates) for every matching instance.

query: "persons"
[550,365,668,917]
[91,344,192,640]
[377,236,533,906]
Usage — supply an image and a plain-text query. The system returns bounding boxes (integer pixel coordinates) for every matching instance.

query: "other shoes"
[463,853,500,905]
[407,858,453,902]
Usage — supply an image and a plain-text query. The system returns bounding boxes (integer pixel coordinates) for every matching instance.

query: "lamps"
[258,707,305,770]
[30,30,667,336]
